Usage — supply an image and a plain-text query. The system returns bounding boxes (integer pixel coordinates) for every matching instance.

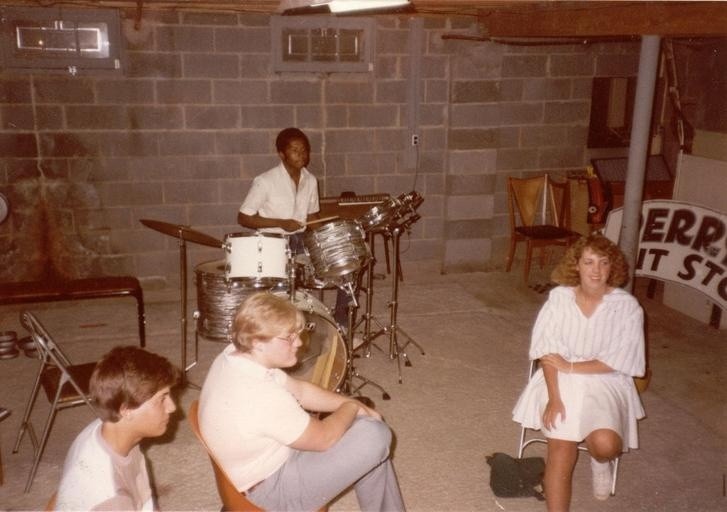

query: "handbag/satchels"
[567,359,574,374]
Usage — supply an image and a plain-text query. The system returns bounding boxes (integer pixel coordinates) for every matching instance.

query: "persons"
[196,291,408,511]
[511,232,646,512]
[53,344,183,511]
[237,128,364,350]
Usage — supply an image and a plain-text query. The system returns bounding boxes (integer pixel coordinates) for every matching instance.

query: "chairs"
[590,455,614,500]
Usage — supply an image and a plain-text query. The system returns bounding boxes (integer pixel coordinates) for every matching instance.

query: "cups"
[274,328,302,345]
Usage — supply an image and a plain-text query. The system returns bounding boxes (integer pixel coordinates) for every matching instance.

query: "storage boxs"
[0,273,147,349]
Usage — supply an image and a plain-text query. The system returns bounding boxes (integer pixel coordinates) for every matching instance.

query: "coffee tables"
[138,219,223,248]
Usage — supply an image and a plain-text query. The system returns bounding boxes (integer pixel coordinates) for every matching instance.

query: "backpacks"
[354,190,423,233]
[193,258,277,341]
[224,230,292,284]
[294,219,371,289]
[271,290,353,395]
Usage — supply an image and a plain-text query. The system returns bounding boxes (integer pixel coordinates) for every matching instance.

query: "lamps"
[518,347,623,499]
[183,395,332,512]
[504,172,582,285]
[10,307,106,493]
